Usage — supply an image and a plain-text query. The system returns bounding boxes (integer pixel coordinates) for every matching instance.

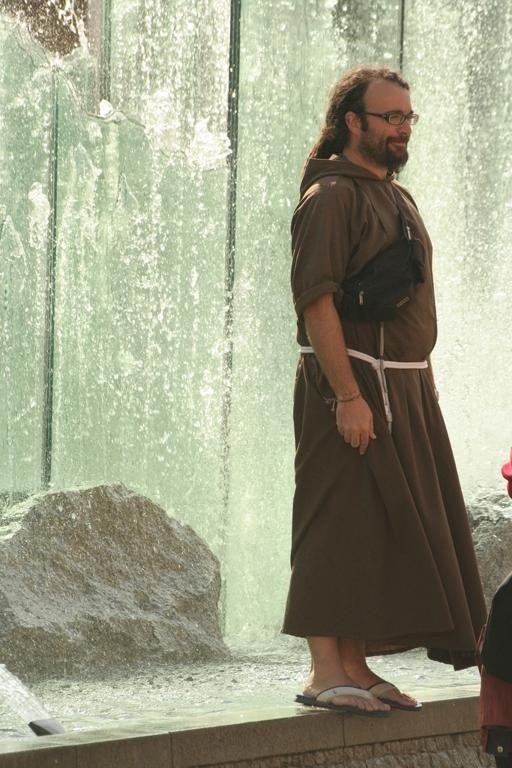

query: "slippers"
[295,680,422,717]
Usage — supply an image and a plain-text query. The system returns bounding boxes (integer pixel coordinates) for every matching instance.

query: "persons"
[480,446,512,768]
[280,64,488,718]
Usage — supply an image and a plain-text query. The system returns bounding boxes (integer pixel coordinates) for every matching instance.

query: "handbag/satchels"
[335,230,426,326]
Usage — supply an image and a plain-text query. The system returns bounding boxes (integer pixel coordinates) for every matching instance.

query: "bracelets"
[336,393,361,403]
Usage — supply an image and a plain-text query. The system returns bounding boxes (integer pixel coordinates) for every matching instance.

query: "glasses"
[365,112,418,125]
